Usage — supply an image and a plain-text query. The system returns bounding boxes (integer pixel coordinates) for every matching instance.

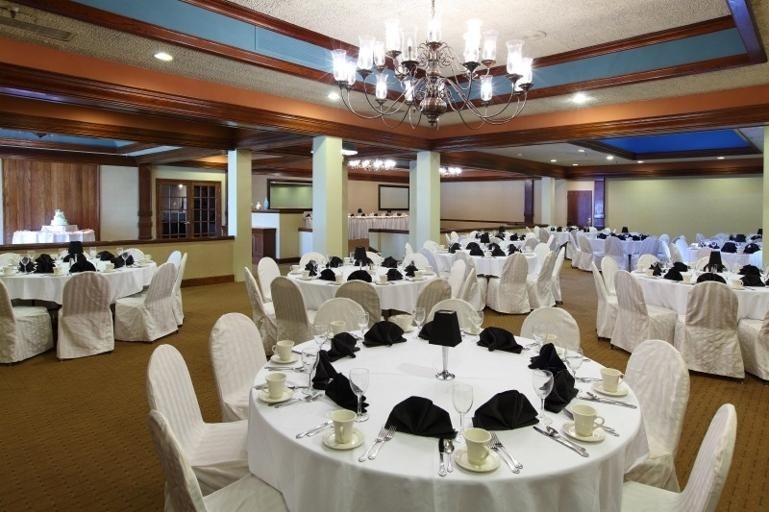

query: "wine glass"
[532,370,555,424]
[700,240,717,250]
[564,344,584,382]
[637,232,643,241]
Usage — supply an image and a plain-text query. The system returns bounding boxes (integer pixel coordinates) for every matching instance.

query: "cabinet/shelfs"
[222,225,228,236]
[251,228,276,265]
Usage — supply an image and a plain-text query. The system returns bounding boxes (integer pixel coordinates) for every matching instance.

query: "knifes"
[533,425,588,458]
[562,407,619,438]
[574,392,637,409]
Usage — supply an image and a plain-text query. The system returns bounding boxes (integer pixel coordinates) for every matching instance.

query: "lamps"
[327,0,537,134]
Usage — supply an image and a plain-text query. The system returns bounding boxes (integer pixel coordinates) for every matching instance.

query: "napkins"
[63,241,96,272]
[96,251,134,269]
[19,254,56,273]
[384,396,458,439]
[311,351,369,413]
[544,369,579,413]
[471,390,539,431]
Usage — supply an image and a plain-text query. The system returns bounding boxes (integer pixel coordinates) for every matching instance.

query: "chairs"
[145,344,248,512]
[115,251,188,344]
[244,226,769,384]
[0,252,22,270]
[623,339,691,493]
[56,271,115,362]
[12,231,95,244]
[621,403,737,512]
[209,312,268,423]
[59,250,92,260]
[0,280,54,365]
[123,248,144,259]
[146,409,287,511]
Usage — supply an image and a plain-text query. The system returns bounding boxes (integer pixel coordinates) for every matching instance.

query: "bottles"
[263,196,269,209]
[255,200,261,210]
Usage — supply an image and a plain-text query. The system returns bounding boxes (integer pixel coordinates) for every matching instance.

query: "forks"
[486,431,524,475]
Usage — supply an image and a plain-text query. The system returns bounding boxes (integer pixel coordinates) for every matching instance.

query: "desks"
[0,259,157,312]
[247,326,649,512]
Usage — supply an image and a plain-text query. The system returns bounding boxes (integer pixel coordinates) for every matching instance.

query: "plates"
[561,422,607,443]
[593,385,630,396]
[452,445,503,473]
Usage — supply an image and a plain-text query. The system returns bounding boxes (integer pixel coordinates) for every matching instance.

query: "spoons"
[575,376,603,383]
[586,391,621,404]
[545,425,586,453]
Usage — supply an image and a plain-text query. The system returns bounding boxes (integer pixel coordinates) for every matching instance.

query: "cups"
[288,256,435,285]
[257,305,486,477]
[0,245,149,277]
[634,257,769,289]
[461,427,492,466]
[600,367,626,393]
[570,404,607,437]
[532,323,558,348]
